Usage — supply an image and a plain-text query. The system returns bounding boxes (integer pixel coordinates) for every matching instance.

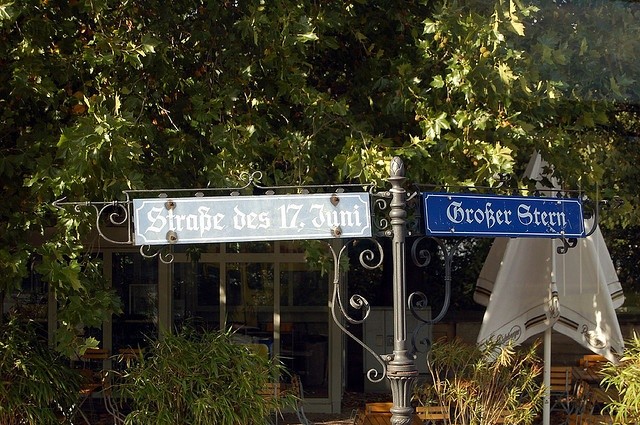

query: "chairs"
[249,381,285,425]
[561,391,596,424]
[98,368,131,425]
[573,365,605,396]
[280,374,312,425]
[550,366,573,416]
[73,348,108,375]
[117,348,146,377]
[415,405,452,424]
[560,379,590,424]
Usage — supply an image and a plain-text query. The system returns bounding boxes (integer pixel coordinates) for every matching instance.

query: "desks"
[45,376,98,424]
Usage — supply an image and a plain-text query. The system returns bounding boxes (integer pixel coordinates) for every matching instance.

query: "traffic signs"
[420,190,588,240]
[133,196,372,249]
